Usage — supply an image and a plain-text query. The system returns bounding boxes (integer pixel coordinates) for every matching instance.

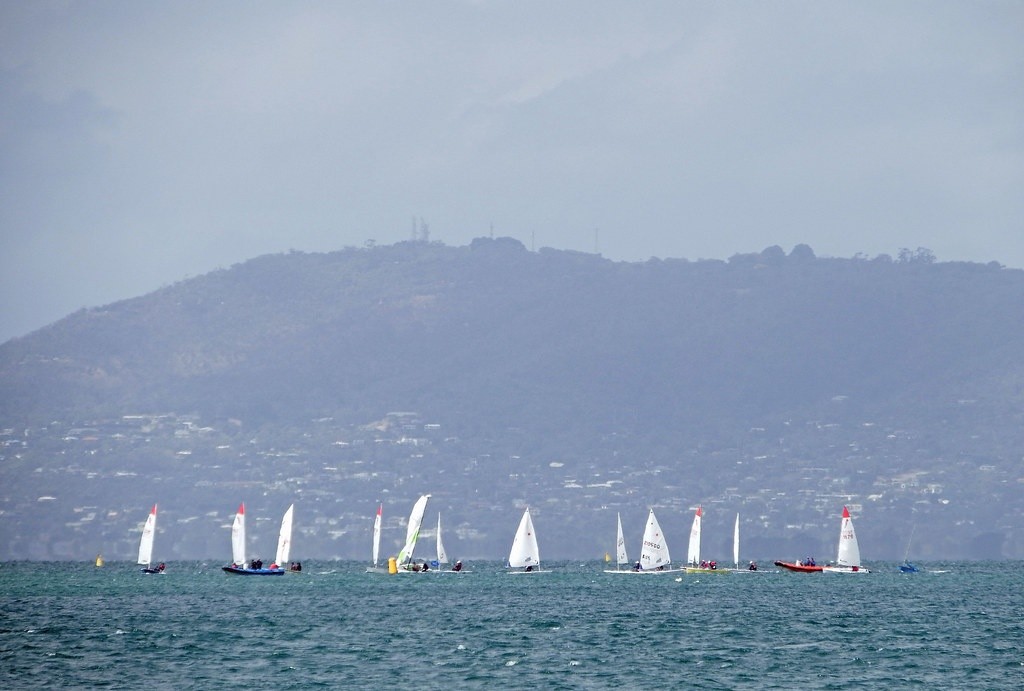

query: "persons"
[412,562,419,571]
[749,561,757,570]
[291,562,301,571]
[700,560,716,570]
[633,561,639,572]
[251,559,262,570]
[422,563,428,572]
[657,566,663,571]
[525,566,533,572]
[270,562,279,569]
[452,561,462,571]
[158,562,165,570]
[805,558,816,566]
[232,563,238,568]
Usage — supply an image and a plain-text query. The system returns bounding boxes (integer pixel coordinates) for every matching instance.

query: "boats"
[896,562,949,574]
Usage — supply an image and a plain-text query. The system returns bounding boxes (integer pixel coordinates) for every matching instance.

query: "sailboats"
[364,494,475,574]
[221,501,294,576]
[682,503,776,574]
[136,503,165,574]
[506,505,554,574]
[601,508,680,574]
[772,503,872,574]
